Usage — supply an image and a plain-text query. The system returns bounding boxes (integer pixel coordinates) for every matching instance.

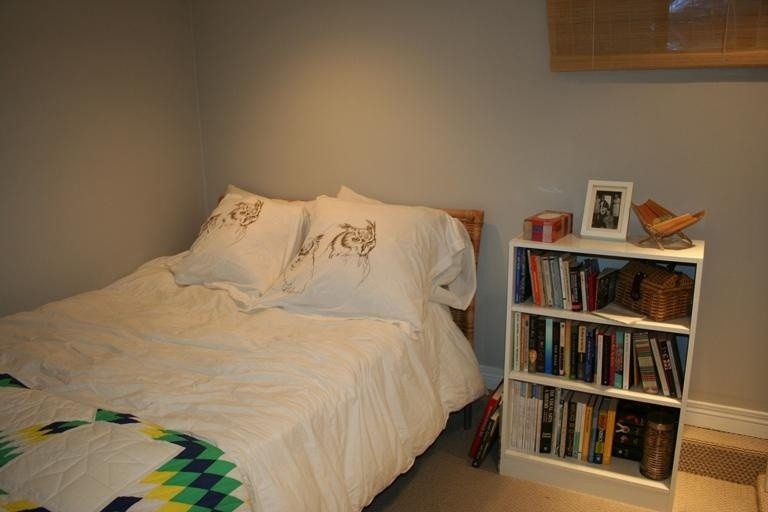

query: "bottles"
[638,416,676,480]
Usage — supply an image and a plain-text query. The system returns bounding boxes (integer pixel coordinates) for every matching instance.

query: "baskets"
[615,258,694,322]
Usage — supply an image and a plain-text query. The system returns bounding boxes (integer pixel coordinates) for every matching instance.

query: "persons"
[592,193,620,229]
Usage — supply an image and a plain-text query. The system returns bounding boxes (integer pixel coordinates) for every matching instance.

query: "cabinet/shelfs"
[495,230,706,512]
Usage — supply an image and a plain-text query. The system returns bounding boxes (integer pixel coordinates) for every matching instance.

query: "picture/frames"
[579,179,634,242]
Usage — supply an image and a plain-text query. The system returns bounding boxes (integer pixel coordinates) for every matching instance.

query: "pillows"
[163,182,308,296]
[259,182,480,326]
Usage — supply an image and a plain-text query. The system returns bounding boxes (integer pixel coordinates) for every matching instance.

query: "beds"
[0,193,486,512]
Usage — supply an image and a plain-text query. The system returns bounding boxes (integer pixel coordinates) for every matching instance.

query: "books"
[508,247,684,465]
[468,373,504,469]
